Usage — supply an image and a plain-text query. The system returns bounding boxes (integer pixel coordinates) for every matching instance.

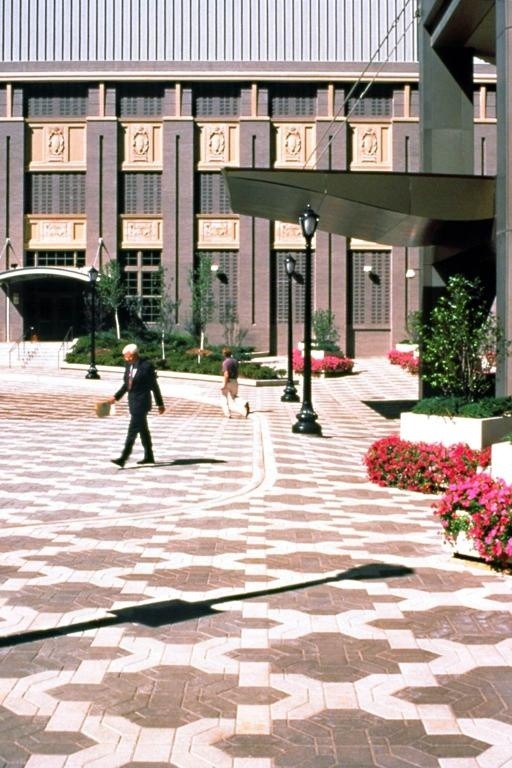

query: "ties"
[127,364,135,390]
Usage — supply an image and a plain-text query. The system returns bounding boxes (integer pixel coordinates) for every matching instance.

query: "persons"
[219,349,250,419]
[107,343,166,468]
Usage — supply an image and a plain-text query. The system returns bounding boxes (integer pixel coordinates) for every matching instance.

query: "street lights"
[291,201,324,436]
[281,252,301,405]
[86,267,102,379]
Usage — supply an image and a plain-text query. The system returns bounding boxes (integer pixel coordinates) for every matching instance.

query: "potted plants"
[399,271,512,453]
[301,306,346,379]
[414,347,421,359]
[297,316,335,352]
[395,308,422,352]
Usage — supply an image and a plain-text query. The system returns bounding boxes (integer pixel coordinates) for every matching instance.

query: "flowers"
[431,469,512,578]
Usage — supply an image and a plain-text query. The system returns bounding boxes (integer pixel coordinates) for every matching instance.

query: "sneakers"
[224,401,250,419]
[110,458,125,468]
[136,458,156,464]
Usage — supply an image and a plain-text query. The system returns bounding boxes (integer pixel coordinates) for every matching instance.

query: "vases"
[451,508,485,562]
